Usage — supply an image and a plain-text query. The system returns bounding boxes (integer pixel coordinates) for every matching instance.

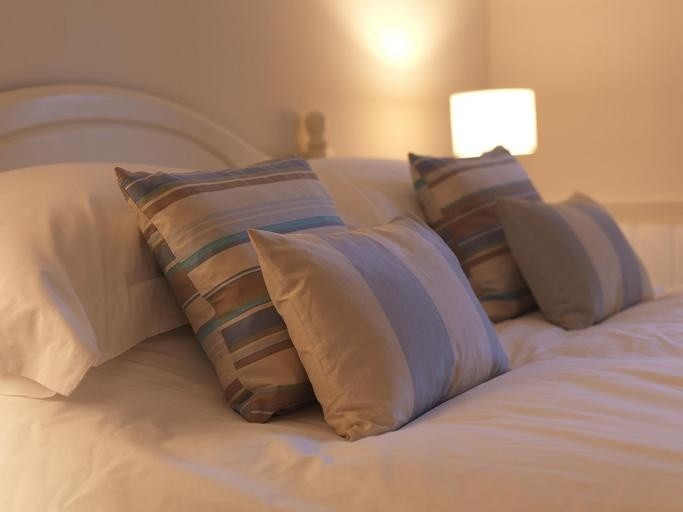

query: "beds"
[0,83,683,512]
